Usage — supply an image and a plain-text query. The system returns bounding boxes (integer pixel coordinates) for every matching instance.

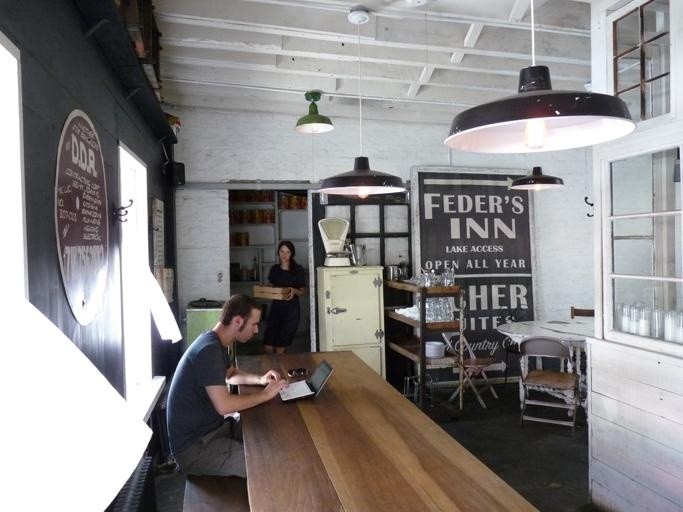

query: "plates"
[425,341,445,358]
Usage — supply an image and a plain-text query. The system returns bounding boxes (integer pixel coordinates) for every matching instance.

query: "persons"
[261,240,305,355]
[164,293,288,478]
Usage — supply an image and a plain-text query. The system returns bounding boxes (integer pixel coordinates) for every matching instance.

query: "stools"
[180,473,246,512]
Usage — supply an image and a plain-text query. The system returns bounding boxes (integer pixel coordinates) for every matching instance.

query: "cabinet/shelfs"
[383,281,465,410]
[229,190,308,287]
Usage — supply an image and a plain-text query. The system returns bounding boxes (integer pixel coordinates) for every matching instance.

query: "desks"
[498,319,597,423]
[234,350,541,512]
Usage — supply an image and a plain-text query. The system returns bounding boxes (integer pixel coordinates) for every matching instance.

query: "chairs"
[439,331,500,410]
[520,335,581,437]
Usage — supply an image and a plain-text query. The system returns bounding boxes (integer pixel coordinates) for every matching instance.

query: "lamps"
[292,6,407,200]
[443,1,637,192]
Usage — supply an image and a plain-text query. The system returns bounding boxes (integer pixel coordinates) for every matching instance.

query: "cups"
[388,266,402,281]
[419,265,455,323]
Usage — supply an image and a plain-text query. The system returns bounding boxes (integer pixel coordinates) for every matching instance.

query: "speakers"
[165,162,185,185]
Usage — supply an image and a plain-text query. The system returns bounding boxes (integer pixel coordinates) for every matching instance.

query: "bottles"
[241,257,259,281]
[229,194,307,224]
[614,301,683,346]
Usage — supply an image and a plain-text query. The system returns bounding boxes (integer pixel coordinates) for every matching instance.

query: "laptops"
[278,358,335,402]
